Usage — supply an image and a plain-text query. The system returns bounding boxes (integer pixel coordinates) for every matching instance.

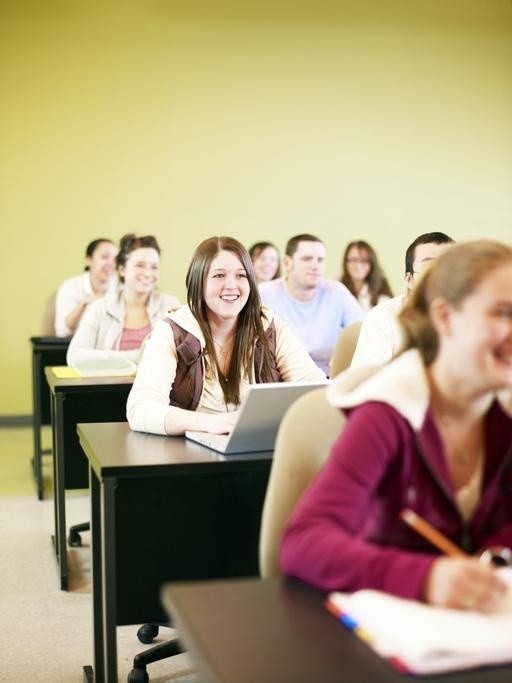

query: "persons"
[126,236,331,435]
[53,240,119,337]
[246,242,281,282]
[339,240,397,309]
[275,241,512,609]
[345,231,460,371]
[257,234,368,376]
[66,235,183,375]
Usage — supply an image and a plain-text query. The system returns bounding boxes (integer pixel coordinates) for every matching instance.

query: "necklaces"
[213,340,235,357]
[440,414,487,468]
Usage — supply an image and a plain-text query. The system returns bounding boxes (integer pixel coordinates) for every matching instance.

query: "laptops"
[185,383,331,455]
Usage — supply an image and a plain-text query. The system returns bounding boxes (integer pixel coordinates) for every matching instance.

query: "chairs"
[259,387,349,580]
[329,321,365,380]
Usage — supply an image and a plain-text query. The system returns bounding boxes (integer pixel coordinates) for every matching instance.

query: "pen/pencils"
[399,507,468,556]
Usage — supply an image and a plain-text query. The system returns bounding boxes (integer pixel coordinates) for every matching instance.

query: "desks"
[44,364,138,592]
[162,575,506,683]
[28,332,75,501]
[73,420,278,682]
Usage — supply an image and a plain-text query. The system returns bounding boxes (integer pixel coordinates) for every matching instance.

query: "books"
[326,560,512,674]
